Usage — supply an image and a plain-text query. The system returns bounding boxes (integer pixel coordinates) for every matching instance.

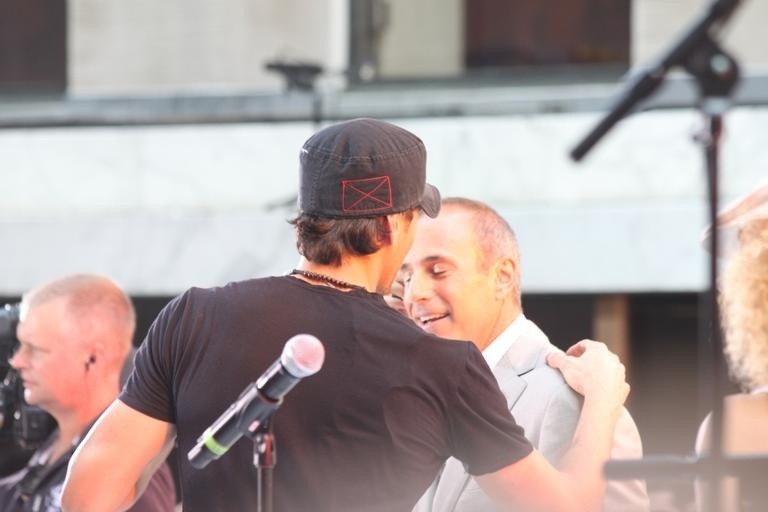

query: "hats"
[299,118,441,219]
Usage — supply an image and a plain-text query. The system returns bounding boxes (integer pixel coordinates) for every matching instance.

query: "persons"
[56,119,630,512]
[12,273,174,512]
[687,197,768,511]
[384,197,651,511]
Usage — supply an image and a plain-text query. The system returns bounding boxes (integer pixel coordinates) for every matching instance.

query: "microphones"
[186,332,326,469]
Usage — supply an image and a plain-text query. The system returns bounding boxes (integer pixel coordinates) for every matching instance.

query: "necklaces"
[290,269,366,291]
